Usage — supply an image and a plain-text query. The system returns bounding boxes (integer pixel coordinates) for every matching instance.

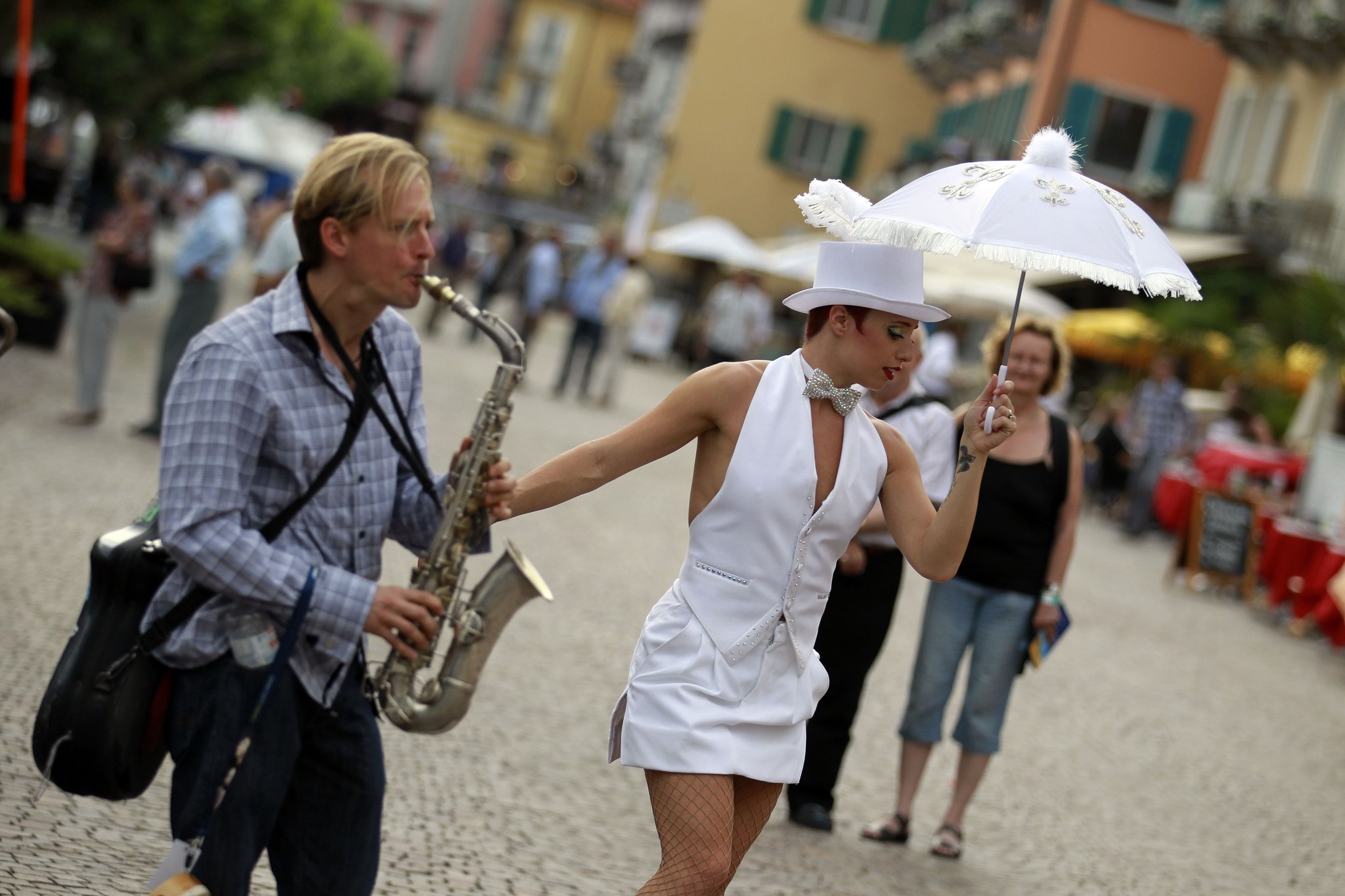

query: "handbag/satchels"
[32,518,170,801]
[109,259,155,301]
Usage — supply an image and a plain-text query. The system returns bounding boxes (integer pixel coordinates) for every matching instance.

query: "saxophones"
[375,273,552,736]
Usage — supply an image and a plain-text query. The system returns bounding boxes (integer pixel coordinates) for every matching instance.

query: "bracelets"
[1038,584,1061,607]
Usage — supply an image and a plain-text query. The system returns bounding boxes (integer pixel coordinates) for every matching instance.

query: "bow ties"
[801,353,862,417]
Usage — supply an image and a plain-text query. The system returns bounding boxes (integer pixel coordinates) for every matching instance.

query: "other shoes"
[788,802,832,832]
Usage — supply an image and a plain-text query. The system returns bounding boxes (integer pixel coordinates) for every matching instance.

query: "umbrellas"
[855,125,1200,434]
[645,216,1070,319]
[159,102,329,180]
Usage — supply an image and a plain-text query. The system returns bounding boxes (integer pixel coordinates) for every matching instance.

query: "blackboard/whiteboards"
[1189,487,1263,584]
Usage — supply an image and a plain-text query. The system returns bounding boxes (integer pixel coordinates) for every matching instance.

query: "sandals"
[861,814,909,844]
[929,824,963,859]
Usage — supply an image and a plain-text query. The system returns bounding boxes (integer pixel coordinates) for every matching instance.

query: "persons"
[1121,351,1188,538]
[57,158,307,436]
[778,323,957,831]
[490,240,1017,896]
[431,221,651,410]
[156,131,518,896]
[699,274,775,365]
[861,313,1082,859]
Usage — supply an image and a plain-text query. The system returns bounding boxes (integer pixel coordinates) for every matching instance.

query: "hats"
[782,241,951,322]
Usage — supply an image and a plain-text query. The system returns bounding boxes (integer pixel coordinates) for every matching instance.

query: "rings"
[1008,409,1012,418]
